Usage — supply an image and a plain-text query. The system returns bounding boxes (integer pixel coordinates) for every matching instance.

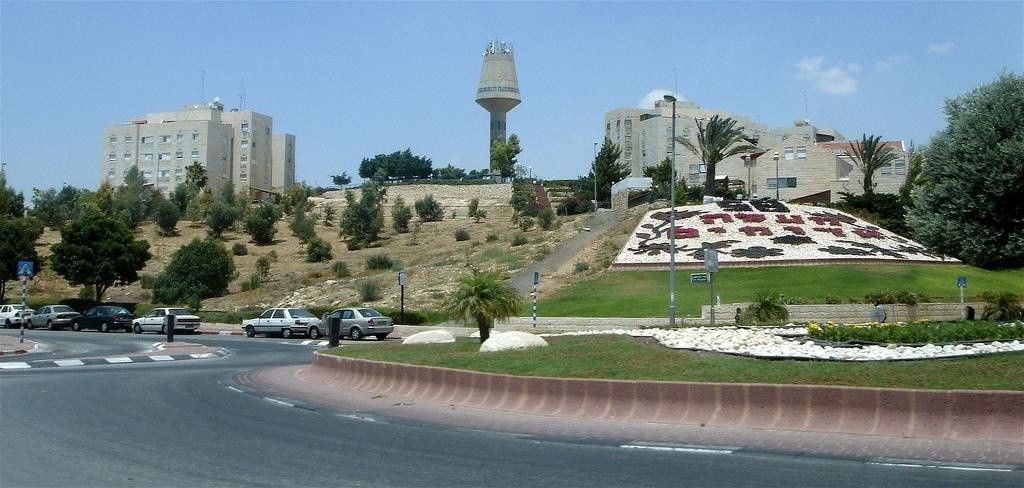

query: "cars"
[240,307,318,339]
[25,304,80,331]
[70,306,137,333]
[130,307,201,335]
[306,307,393,342]
[0,303,35,328]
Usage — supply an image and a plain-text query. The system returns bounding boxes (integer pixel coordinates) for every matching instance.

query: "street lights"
[398,272,406,326]
[529,166,532,179]
[662,93,678,324]
[592,142,597,210]
[773,151,779,200]
[0,162,6,169]
[744,154,750,197]
